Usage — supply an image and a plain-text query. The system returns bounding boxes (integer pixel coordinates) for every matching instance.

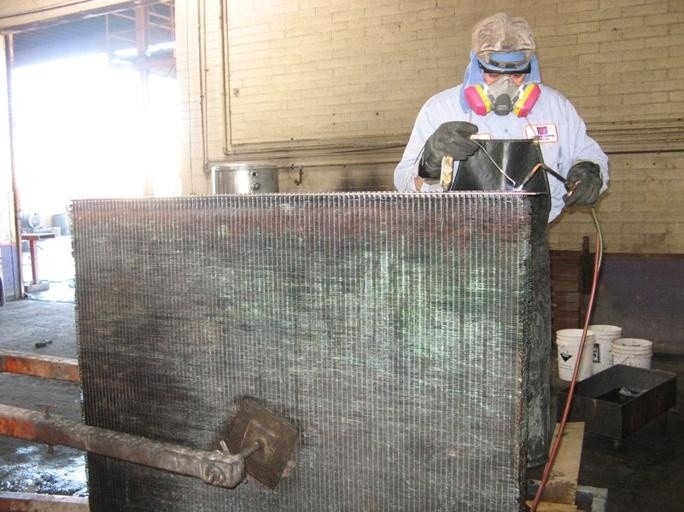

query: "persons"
[392,13,610,223]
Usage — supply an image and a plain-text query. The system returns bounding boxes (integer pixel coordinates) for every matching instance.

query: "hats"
[473,12,536,70]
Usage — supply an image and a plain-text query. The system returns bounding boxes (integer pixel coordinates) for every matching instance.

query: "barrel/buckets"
[610,337,653,372]
[556,328,594,382]
[586,325,623,372]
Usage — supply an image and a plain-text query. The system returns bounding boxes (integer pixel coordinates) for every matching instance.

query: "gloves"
[563,161,603,207]
[420,120,480,177]
